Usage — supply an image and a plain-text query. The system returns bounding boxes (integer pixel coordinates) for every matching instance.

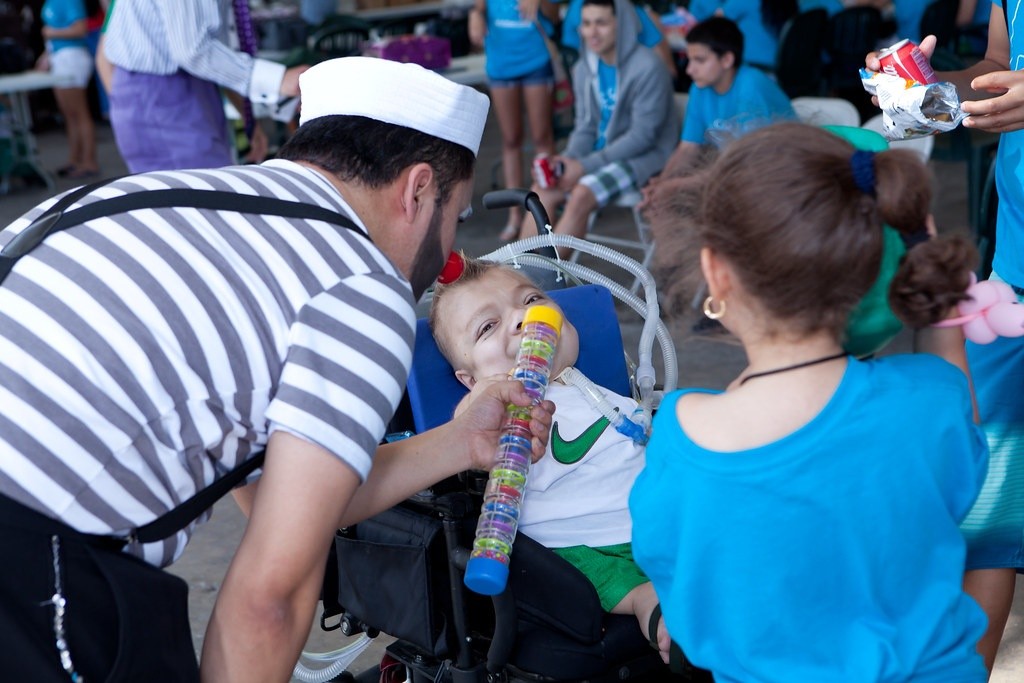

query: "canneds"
[532,152,557,189]
[876,38,939,86]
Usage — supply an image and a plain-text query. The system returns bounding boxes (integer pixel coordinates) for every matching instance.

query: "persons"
[1,57,490,683]
[625,122,991,683]
[868,2,1024,683]
[0,0,999,335]
[432,257,677,664]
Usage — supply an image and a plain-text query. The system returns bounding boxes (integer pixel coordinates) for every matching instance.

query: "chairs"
[867,112,935,162]
[308,15,369,53]
[790,98,860,129]
[564,94,693,300]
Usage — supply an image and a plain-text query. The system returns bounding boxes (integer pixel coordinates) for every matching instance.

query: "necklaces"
[735,349,850,386]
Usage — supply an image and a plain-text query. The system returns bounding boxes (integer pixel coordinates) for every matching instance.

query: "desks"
[349,6,442,41]
[439,53,490,86]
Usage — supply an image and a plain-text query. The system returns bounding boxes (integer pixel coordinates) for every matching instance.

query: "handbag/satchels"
[361,25,452,68]
[315,495,451,652]
[553,78,574,116]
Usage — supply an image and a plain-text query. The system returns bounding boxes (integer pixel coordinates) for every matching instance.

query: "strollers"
[319,188,715,683]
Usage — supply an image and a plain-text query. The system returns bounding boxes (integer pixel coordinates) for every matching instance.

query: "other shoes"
[638,265,685,322]
[693,315,724,335]
[500,217,522,241]
[57,158,101,180]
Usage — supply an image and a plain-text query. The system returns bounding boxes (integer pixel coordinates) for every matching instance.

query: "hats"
[298,56,490,158]
